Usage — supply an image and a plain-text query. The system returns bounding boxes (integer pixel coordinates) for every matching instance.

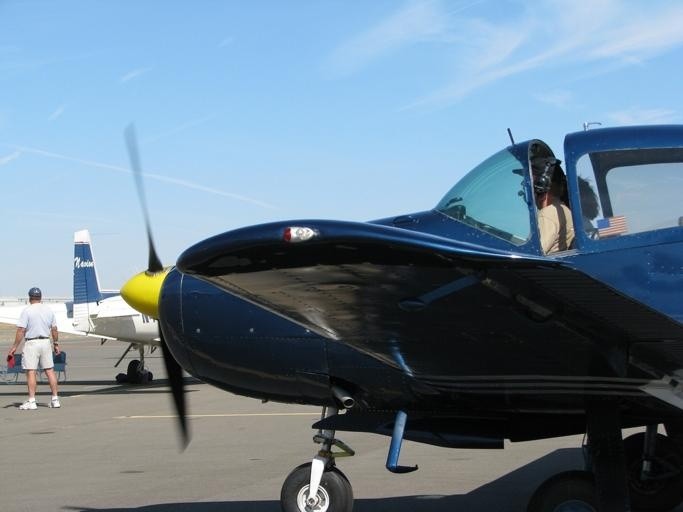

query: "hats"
[512,157,564,186]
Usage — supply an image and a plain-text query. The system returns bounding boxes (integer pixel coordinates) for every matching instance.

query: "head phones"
[534,157,561,194]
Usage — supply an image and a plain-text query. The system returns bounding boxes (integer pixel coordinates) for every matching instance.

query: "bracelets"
[53,342,59,345]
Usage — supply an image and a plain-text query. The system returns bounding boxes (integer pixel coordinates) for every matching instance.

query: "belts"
[26,336,49,340]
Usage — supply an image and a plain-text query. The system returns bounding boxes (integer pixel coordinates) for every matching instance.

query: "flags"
[596,215,629,238]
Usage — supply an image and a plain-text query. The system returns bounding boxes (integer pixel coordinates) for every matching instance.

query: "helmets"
[28,287,41,297]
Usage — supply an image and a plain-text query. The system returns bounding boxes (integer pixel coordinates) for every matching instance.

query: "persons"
[8,287,60,410]
[519,155,597,256]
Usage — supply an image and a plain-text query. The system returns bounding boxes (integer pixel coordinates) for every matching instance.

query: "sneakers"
[19,401,37,410]
[47,399,60,408]
